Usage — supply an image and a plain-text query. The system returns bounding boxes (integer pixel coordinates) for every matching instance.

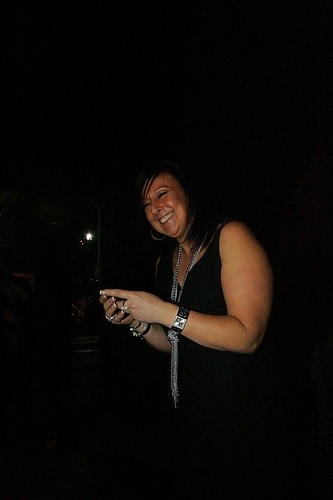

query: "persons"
[98,165,274,500]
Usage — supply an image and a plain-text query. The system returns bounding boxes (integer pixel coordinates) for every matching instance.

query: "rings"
[105,314,114,320]
[113,316,120,324]
[121,300,128,312]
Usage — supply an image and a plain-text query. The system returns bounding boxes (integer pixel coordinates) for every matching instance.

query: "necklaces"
[171,244,201,407]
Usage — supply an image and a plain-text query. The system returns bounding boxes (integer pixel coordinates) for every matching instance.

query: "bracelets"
[130,322,152,339]
[168,307,190,338]
